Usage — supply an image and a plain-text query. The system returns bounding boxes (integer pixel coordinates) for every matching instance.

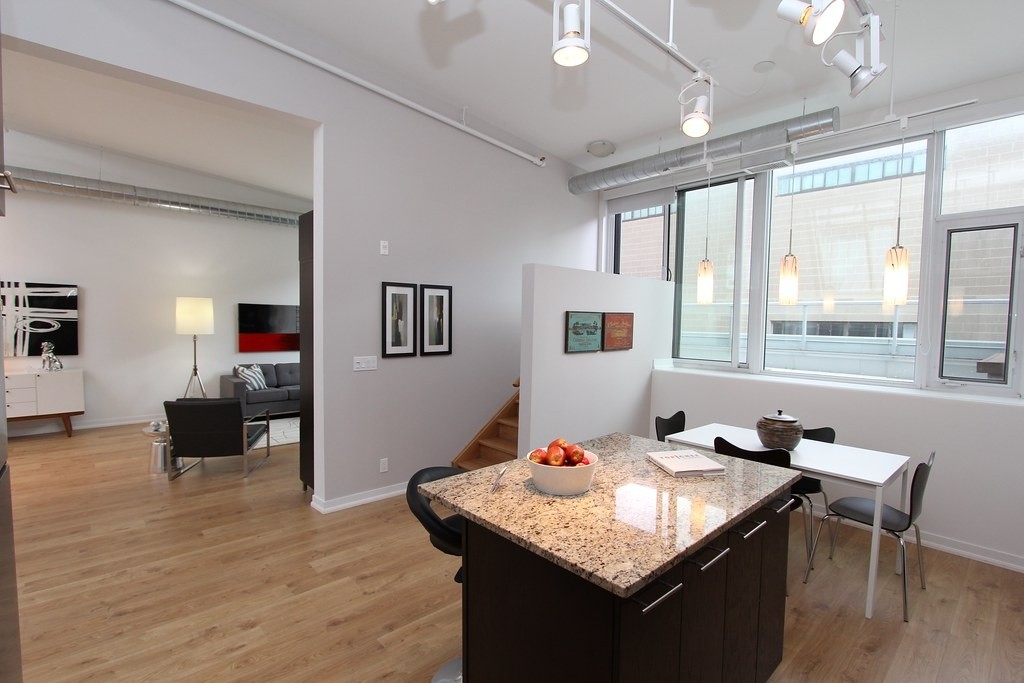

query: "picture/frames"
[420,284,453,356]
[566,311,603,352]
[382,282,417,357]
[603,312,634,351]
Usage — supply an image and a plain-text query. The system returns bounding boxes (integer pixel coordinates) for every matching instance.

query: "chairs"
[791,426,833,550]
[163,398,271,481]
[804,450,936,622]
[714,437,814,572]
[655,410,685,442]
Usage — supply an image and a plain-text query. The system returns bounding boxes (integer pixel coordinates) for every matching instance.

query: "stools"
[406,467,468,683]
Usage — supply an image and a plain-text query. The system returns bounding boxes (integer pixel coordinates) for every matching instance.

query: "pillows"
[234,363,260,375]
[239,369,267,391]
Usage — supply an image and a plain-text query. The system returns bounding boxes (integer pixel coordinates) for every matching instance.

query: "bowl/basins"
[527,447,599,496]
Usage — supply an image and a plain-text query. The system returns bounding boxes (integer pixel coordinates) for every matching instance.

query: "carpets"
[247,417,300,450]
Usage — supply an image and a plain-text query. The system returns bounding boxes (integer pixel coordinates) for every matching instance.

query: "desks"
[665,422,911,619]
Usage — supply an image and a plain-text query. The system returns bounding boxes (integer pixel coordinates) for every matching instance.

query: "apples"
[530,439,591,467]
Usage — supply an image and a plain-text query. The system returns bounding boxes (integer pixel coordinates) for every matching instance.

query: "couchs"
[220,363,300,421]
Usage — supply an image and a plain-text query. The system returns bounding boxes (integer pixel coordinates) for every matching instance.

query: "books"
[646,450,726,478]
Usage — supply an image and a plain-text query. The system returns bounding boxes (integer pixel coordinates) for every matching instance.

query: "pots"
[756,410,803,450]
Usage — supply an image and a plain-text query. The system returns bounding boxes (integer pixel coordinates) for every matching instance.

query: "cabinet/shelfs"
[4,370,85,437]
[418,489,804,683]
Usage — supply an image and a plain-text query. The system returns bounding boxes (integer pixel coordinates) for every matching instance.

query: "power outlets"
[379,458,388,473]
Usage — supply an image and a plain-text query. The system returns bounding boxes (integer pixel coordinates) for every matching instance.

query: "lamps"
[665,0,979,305]
[175,296,215,398]
[587,141,615,158]
[552,0,718,139]
